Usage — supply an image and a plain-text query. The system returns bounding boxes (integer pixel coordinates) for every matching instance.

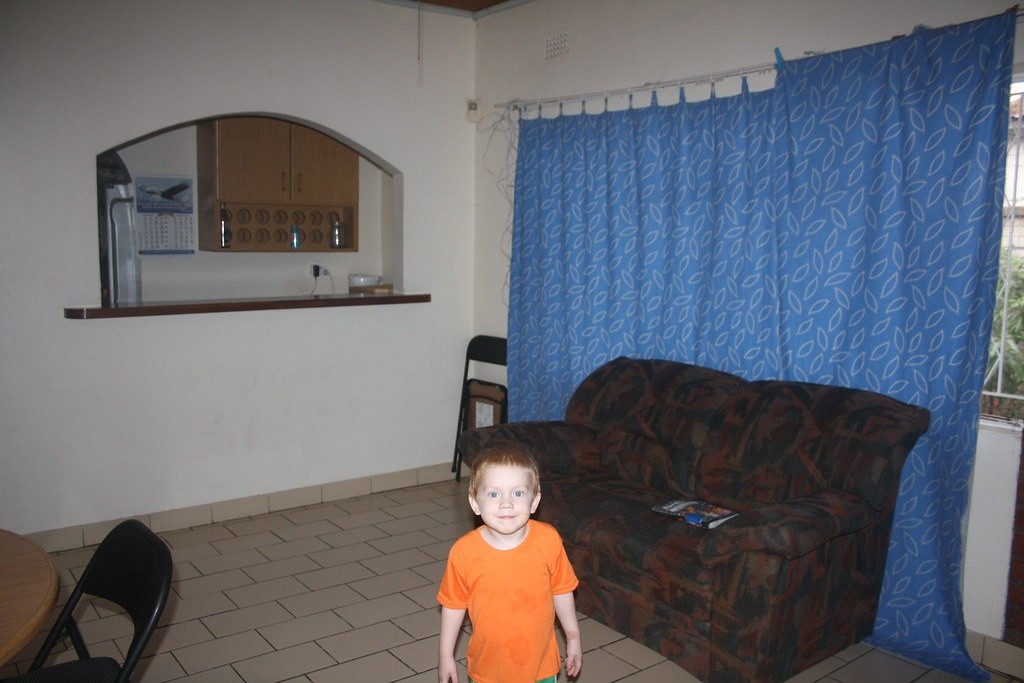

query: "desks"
[0,528,62,670]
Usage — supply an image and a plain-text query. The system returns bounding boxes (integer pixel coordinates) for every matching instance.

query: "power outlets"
[309,262,328,278]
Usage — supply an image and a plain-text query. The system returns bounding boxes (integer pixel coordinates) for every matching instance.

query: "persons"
[436,444,583,683]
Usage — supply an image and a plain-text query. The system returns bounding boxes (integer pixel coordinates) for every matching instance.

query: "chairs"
[451,334,511,481]
[0,518,173,683]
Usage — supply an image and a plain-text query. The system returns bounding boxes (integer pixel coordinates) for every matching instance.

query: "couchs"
[456,355,931,683]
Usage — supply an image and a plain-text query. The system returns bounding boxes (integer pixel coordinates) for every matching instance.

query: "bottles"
[331,221,344,248]
[220,202,229,248]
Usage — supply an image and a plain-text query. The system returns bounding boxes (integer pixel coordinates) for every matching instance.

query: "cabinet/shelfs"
[196,119,360,254]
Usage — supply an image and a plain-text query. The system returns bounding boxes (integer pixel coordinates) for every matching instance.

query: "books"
[652,497,740,530]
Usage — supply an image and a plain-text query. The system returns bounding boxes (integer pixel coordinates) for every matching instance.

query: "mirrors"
[95,110,408,312]
